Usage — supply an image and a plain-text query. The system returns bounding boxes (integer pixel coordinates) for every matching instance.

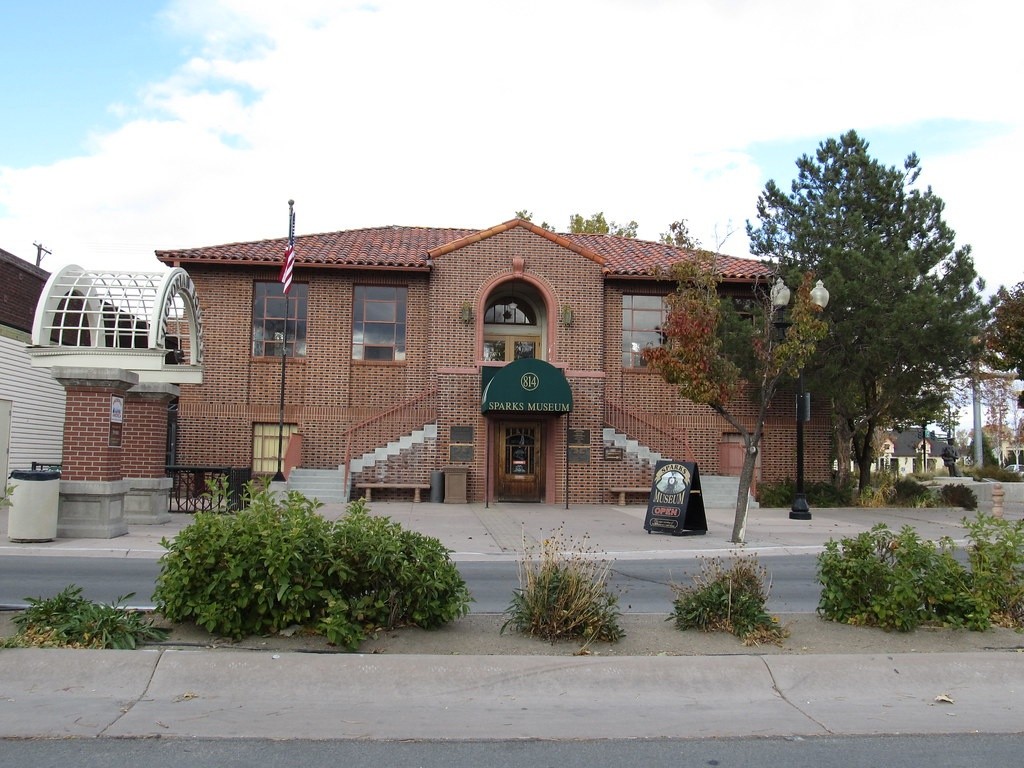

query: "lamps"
[561,305,573,328]
[460,300,473,324]
[508,301,518,310]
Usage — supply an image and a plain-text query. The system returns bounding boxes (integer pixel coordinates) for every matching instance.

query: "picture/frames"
[567,428,591,446]
[448,444,476,463]
[604,447,623,461]
[567,446,592,465]
[448,425,474,443]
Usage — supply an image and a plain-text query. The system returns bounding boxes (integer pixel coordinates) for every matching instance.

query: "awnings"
[480,357,573,510]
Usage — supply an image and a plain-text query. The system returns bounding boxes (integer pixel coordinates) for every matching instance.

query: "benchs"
[355,482,430,503]
[608,485,700,507]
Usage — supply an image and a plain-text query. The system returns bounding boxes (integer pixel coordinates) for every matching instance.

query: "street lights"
[772,278,830,519]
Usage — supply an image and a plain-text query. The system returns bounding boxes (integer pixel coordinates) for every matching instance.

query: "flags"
[278,212,296,296]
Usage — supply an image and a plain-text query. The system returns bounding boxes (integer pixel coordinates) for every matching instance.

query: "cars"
[1004,464,1024,478]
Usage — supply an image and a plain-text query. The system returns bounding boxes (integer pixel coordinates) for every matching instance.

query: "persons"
[941,438,960,477]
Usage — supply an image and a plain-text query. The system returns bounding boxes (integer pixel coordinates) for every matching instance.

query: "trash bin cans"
[7,469,61,544]
